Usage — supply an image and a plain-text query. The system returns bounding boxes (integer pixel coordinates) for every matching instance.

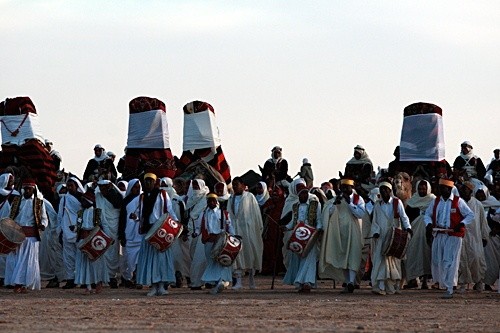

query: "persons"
[0,138,500,299]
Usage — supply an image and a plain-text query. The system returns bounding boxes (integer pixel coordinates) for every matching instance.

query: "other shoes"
[15,276,255,296]
[421,283,429,289]
[299,285,310,293]
[442,293,453,298]
[347,283,353,292]
[404,282,418,289]
[485,286,498,292]
[432,283,439,288]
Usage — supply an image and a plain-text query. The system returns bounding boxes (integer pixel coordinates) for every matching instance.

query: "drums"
[0,217,26,255]
[209,233,242,267]
[78,226,114,263]
[381,227,410,260]
[145,213,183,253]
[286,221,318,257]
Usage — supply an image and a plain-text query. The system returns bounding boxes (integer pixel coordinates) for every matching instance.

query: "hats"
[144,173,157,181]
[439,178,454,187]
[341,179,354,185]
[379,182,392,190]
[462,182,474,190]
[207,194,217,199]
